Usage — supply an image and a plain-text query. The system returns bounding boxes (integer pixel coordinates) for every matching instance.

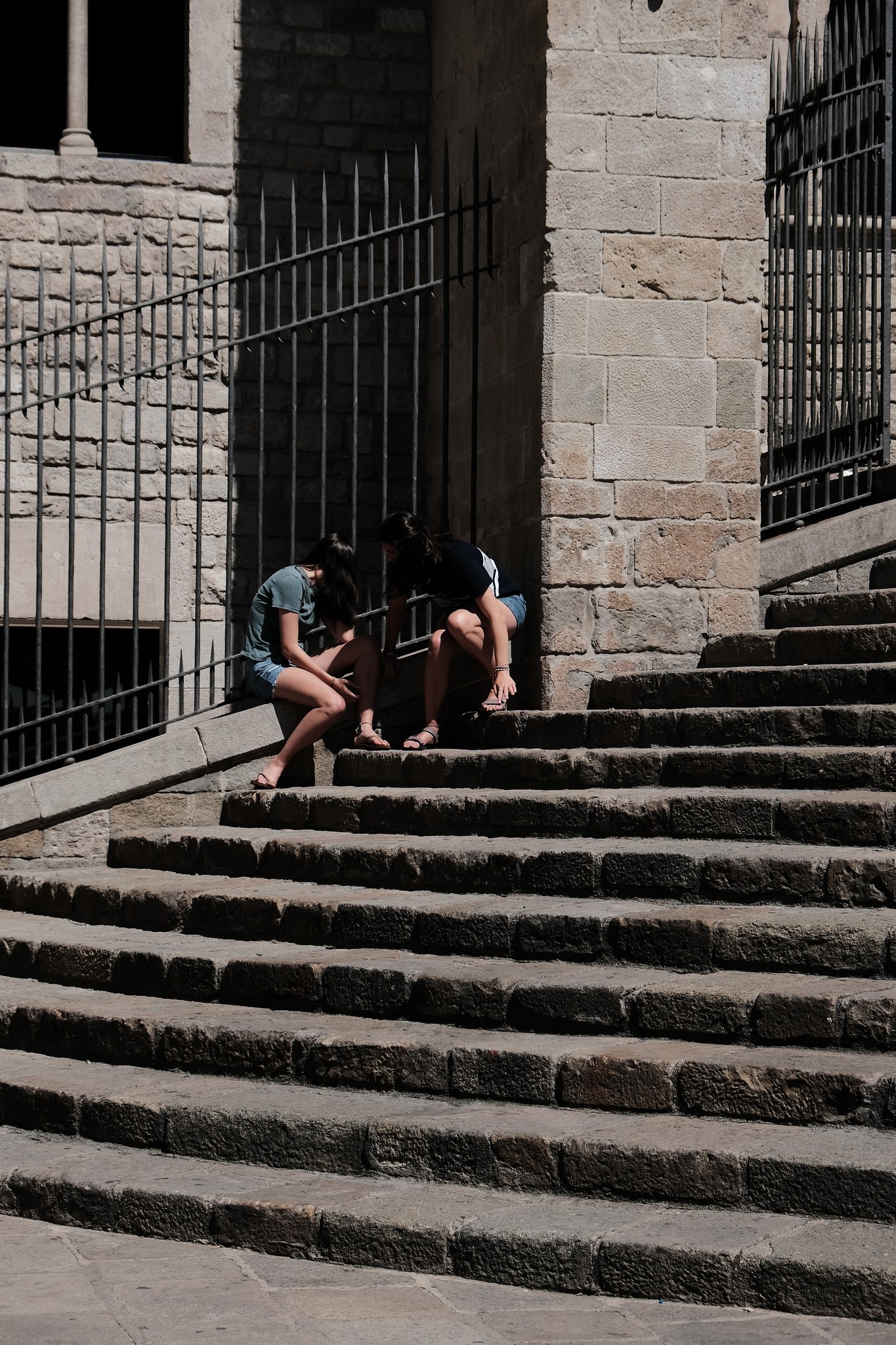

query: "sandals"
[402,727,439,751]
[478,690,509,715]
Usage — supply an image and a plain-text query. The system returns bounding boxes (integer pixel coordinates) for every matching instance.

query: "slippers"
[250,773,277,789]
[351,735,391,750]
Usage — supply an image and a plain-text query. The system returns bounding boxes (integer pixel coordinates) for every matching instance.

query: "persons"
[240,533,391,790]
[377,510,526,751]
